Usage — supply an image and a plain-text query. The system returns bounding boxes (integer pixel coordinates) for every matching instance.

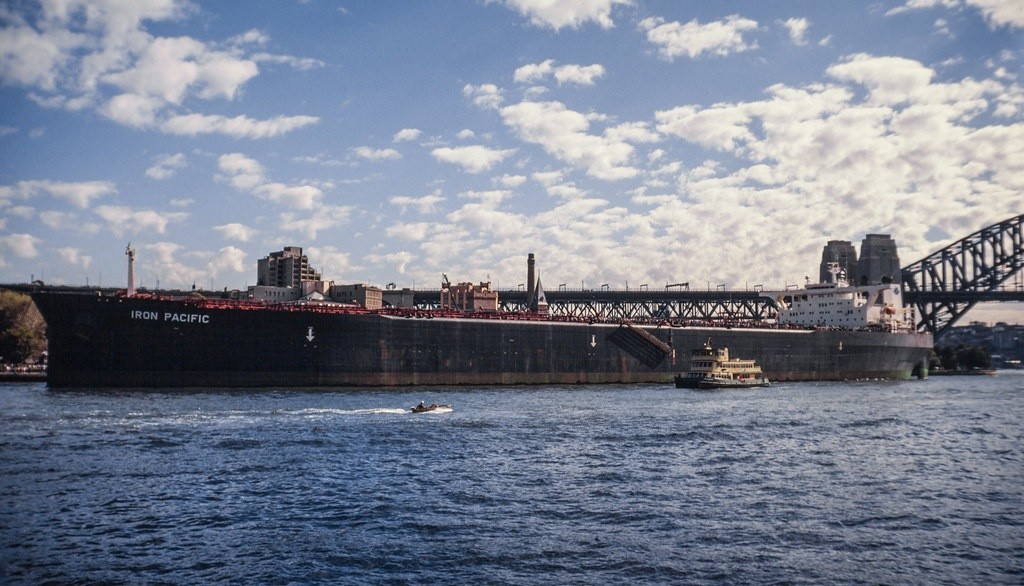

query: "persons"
[3,361,47,373]
[416,400,425,409]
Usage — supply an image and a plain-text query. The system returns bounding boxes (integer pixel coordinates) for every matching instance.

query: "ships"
[23,244,934,389]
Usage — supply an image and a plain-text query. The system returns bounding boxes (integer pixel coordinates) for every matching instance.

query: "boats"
[410,402,453,415]
[673,337,769,387]
